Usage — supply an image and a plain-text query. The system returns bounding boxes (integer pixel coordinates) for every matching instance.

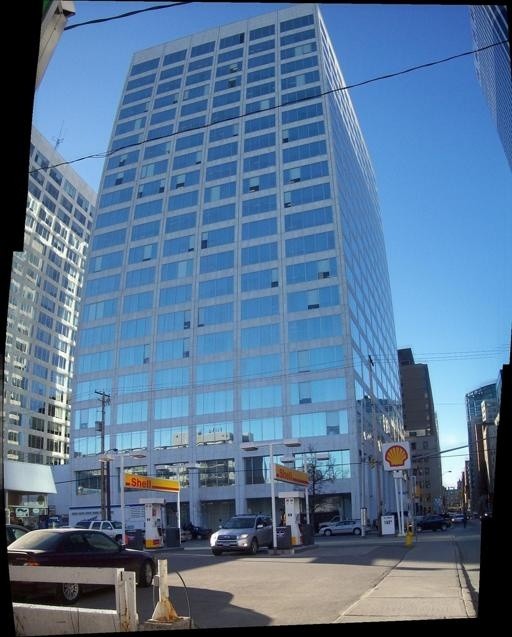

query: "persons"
[35,495,46,507]
[216,518,224,531]
[279,511,286,527]
[463,512,468,529]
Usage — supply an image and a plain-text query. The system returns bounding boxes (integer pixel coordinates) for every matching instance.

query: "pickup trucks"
[72,521,137,544]
[374,511,425,530]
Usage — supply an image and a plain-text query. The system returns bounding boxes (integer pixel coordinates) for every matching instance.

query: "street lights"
[97,450,146,551]
[240,439,303,555]
[279,453,330,525]
[106,447,118,519]
[359,396,372,537]
[155,463,201,543]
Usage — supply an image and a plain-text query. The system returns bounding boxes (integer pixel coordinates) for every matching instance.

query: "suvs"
[319,515,343,528]
[210,511,275,555]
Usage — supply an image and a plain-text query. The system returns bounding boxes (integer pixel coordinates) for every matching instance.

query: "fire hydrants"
[405,531,415,548]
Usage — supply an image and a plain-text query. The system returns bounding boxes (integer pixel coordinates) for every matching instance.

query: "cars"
[181,522,212,540]
[163,526,193,541]
[5,524,29,544]
[319,519,373,535]
[408,514,452,531]
[7,526,158,601]
[447,512,465,522]
